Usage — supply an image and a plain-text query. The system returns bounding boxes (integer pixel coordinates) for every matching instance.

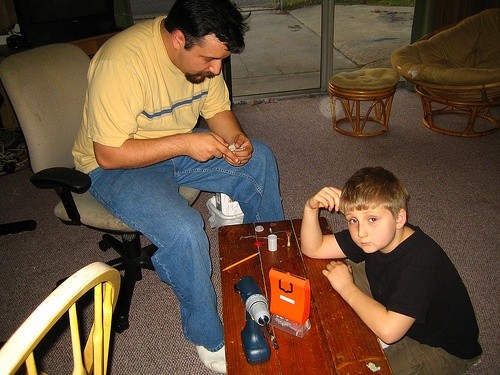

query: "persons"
[73,1,284,375]
[299,165,484,375]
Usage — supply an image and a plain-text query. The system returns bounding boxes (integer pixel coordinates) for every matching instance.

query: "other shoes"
[193,337,228,373]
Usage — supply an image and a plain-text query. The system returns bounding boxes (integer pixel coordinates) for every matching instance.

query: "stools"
[329,67,400,138]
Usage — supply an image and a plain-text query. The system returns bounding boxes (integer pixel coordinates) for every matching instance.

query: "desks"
[218,218,392,375]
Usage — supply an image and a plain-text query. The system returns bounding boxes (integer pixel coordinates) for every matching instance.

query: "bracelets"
[309,200,319,209]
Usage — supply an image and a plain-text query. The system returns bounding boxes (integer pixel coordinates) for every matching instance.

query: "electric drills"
[234,275,279,364]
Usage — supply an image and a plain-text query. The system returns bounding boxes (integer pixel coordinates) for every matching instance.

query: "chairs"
[392,8,500,137]
[0,43,200,330]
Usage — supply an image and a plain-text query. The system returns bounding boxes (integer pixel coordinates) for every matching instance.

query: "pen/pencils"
[223,253,259,271]
